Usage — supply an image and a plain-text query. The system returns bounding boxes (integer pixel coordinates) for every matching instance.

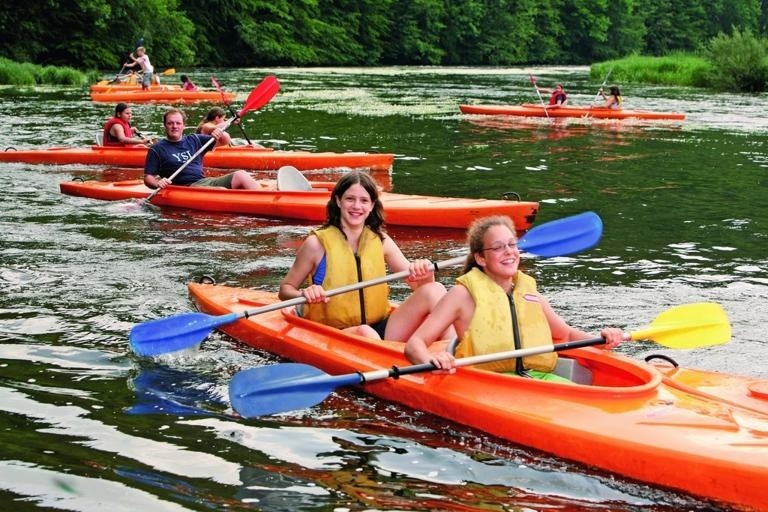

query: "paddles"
[108,38,144,84]
[212,78,252,144]
[228,301,732,418]
[130,212,604,357]
[159,69,175,75]
[530,73,550,119]
[147,76,281,201]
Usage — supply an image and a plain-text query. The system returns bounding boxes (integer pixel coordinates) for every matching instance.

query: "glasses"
[480,243,518,253]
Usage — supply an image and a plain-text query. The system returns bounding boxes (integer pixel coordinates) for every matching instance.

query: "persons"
[195,105,241,148]
[535,84,568,110]
[125,70,137,85]
[179,75,197,91]
[123,46,154,90]
[275,169,458,345]
[142,109,278,192]
[403,212,626,387]
[102,103,153,148]
[152,71,160,86]
[591,119,627,145]
[590,85,623,110]
[546,117,571,140]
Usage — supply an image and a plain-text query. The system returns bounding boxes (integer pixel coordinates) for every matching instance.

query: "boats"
[172,272,768,512]
[456,100,685,121]
